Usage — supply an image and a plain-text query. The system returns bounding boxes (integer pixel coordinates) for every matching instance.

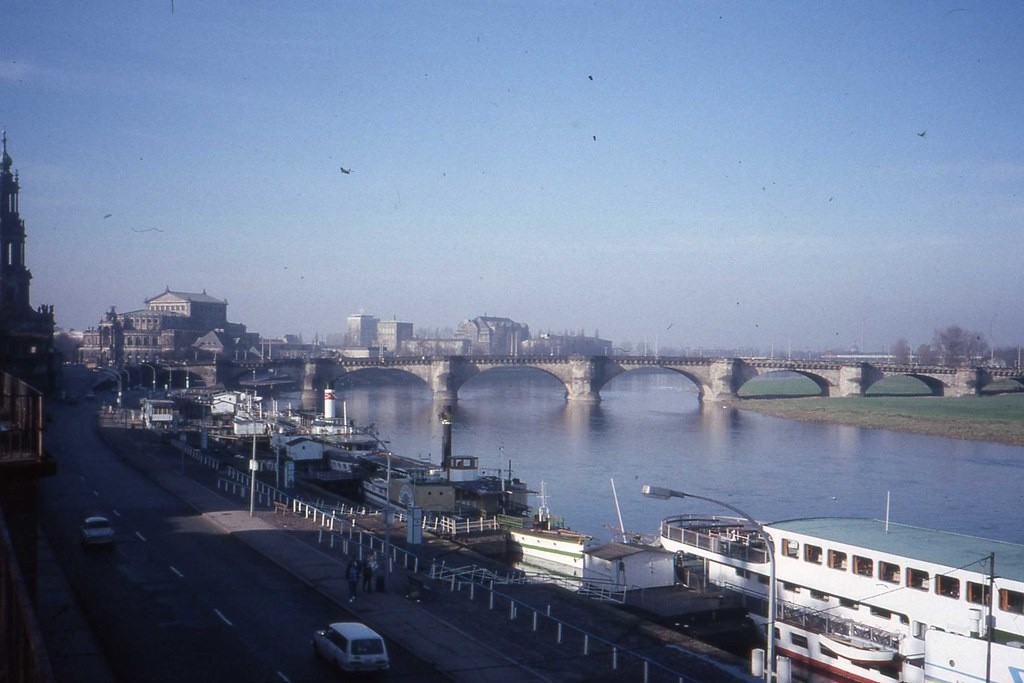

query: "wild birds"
[340,167,355,174]
[916,129,929,139]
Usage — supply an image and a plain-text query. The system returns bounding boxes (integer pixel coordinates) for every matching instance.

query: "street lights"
[110,364,130,388]
[94,365,122,409]
[174,362,190,389]
[310,420,391,573]
[157,362,172,394]
[139,362,156,393]
[642,486,776,683]
[197,396,259,518]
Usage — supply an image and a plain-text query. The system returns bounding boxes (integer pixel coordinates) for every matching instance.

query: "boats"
[657,490,1024,683]
[818,633,896,667]
[165,389,755,654]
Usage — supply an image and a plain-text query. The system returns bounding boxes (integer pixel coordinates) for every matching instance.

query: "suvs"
[314,622,392,674]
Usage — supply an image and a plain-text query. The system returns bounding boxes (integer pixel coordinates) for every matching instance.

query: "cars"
[82,518,115,546]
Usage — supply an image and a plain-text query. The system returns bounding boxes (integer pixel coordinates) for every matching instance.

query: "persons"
[346,560,386,591]
